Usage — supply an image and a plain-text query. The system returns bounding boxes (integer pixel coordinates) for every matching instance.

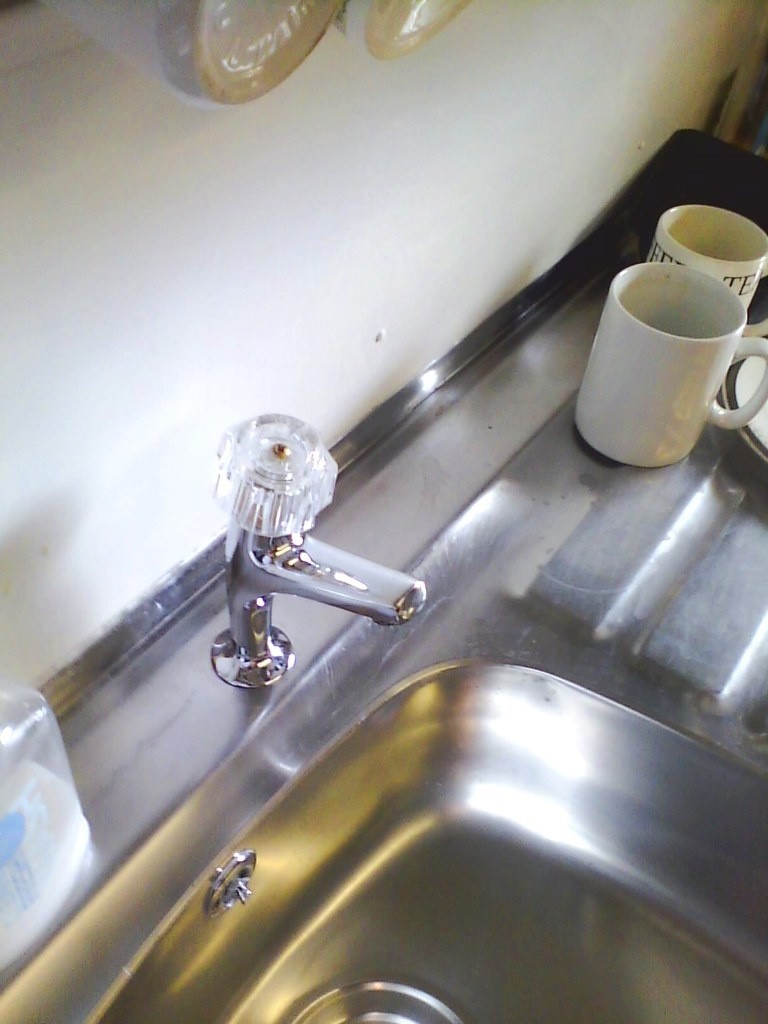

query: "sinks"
[0,636,768,1024]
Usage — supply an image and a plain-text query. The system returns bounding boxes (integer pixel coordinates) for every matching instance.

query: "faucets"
[207,409,429,691]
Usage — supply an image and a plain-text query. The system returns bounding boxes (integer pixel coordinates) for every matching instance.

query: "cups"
[646,204,768,308]
[574,261,768,467]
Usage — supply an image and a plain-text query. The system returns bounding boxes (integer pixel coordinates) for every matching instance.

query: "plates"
[722,356,768,463]
[199,0,338,106]
[364,0,469,61]
